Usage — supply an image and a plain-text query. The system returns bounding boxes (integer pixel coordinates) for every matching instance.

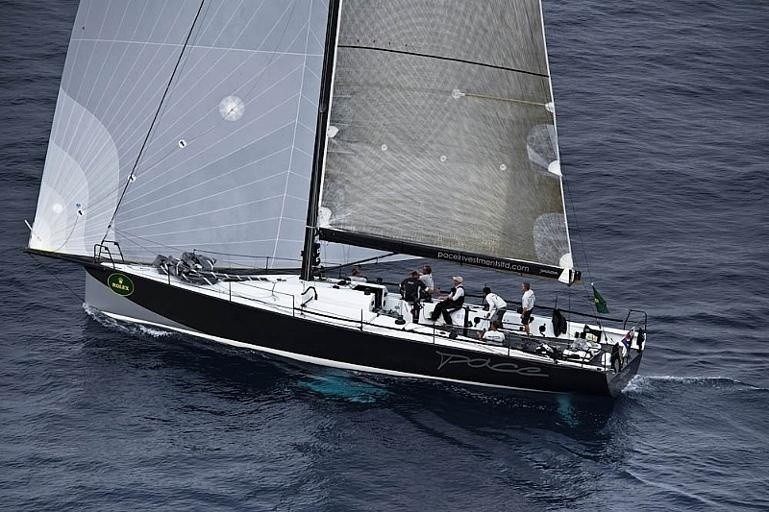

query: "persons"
[418,265,436,292]
[482,288,509,331]
[399,271,428,302]
[427,277,467,326]
[521,281,537,338]
[351,268,361,276]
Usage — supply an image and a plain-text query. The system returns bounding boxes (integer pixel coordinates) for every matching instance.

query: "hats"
[453,276,463,282]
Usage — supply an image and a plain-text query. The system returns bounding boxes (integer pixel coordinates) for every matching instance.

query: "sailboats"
[24,0,647,406]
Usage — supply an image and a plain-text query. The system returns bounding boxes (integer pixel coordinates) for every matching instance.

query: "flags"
[592,286,612,314]
[622,326,636,359]
